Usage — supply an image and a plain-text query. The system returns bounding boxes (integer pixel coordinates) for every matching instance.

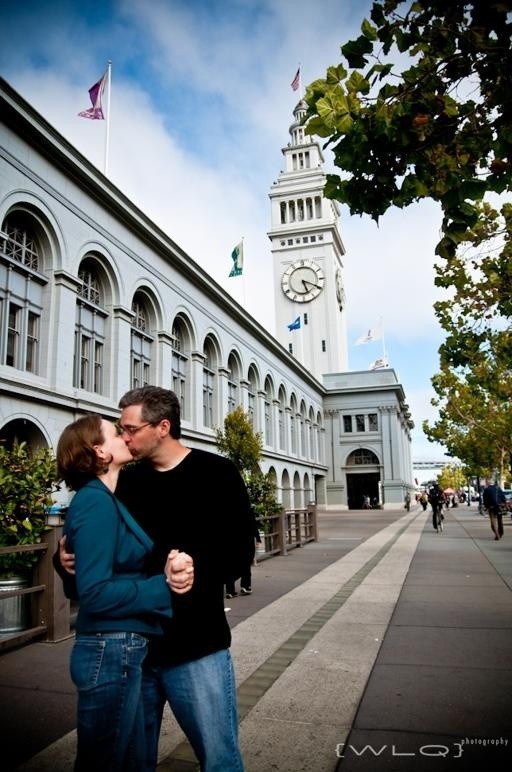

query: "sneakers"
[226,592,239,597]
[241,588,252,594]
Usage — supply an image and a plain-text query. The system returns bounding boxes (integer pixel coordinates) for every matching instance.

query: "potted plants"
[1,438,63,643]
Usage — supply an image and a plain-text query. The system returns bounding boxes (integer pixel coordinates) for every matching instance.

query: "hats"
[432,481,440,485]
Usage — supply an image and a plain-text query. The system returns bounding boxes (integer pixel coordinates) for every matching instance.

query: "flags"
[354,318,384,344]
[228,242,243,278]
[291,68,302,93]
[288,316,301,332]
[79,70,109,120]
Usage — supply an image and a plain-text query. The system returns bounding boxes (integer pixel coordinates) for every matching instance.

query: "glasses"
[120,423,150,434]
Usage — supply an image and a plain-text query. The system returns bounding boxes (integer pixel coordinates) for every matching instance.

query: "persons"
[54,384,264,772]
[226,501,261,599]
[53,414,193,769]
[402,476,510,542]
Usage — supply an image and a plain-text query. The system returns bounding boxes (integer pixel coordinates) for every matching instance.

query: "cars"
[501,488,512,512]
[458,486,480,502]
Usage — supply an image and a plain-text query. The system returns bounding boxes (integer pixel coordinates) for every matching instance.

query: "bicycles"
[430,498,445,533]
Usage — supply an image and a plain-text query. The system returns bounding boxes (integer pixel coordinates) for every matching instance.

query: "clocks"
[281,259,324,302]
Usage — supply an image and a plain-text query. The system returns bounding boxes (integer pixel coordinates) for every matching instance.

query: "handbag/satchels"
[498,503,507,514]
